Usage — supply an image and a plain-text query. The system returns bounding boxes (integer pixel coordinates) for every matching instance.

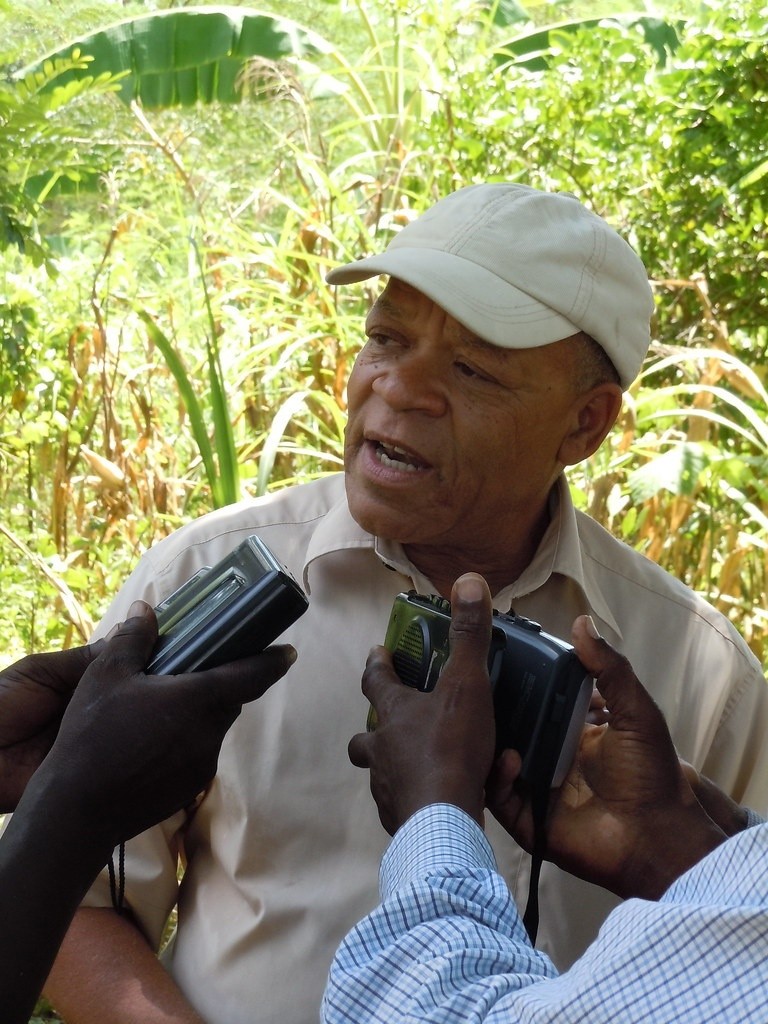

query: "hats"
[325,184,652,395]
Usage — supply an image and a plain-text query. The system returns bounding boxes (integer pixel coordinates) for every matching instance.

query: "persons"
[35,180,768,1024]
[317,573,768,1024]
[0,599,298,1024]
